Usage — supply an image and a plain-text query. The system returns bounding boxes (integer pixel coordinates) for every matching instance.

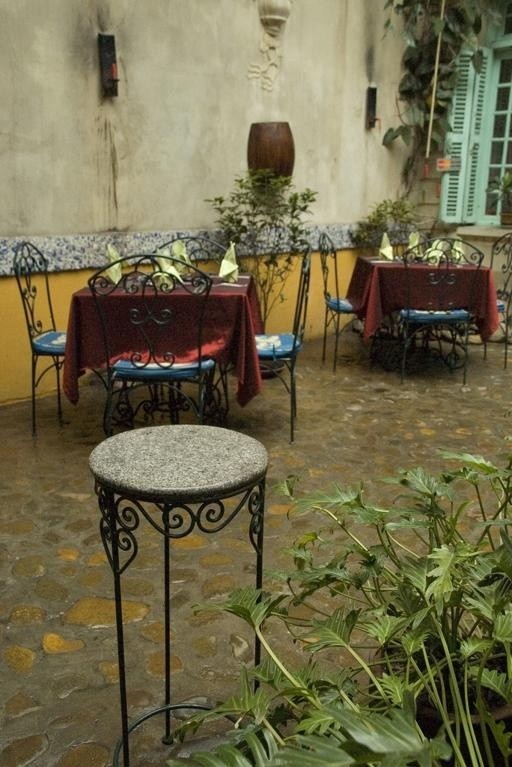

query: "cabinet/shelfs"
[88,425,269,767]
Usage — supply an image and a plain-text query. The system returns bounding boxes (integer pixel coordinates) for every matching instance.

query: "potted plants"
[262,447,512,767]
[485,172,512,224]
